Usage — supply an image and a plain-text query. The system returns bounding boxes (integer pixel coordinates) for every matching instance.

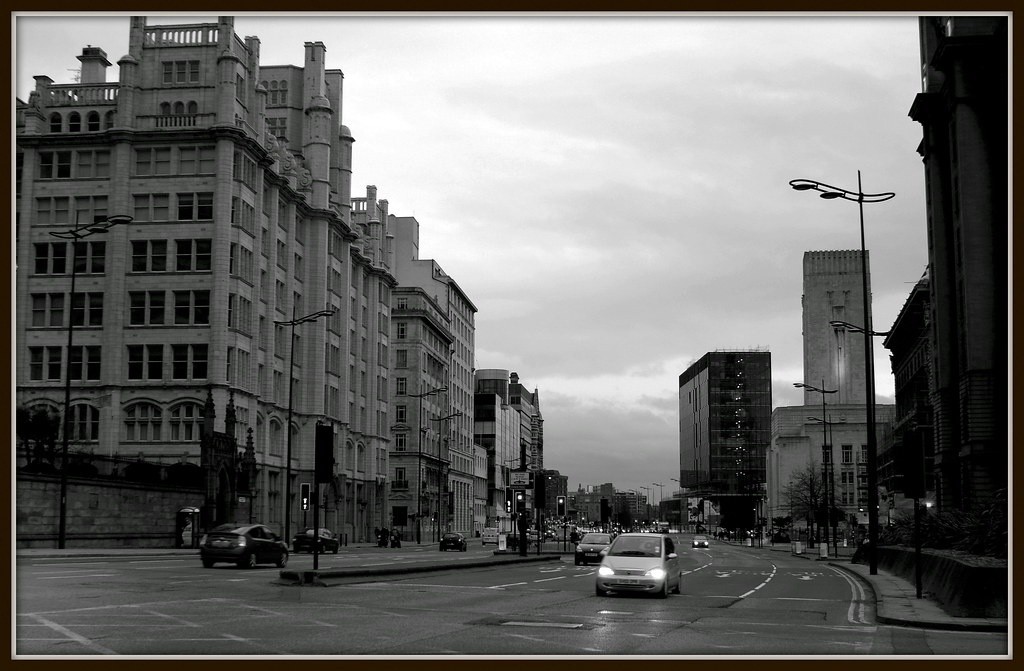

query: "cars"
[506,518,658,566]
[596,533,681,598]
[200,523,290,569]
[293,527,338,554]
[439,533,467,552]
[692,536,708,548]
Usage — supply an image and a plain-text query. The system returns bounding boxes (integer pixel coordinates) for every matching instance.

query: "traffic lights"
[300,482,310,511]
[557,495,566,516]
[505,486,514,514]
[515,492,525,512]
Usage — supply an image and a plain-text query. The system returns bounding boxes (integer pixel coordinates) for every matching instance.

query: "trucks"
[657,522,670,533]
[482,528,500,545]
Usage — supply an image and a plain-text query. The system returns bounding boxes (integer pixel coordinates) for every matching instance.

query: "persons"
[374,526,401,548]
[571,528,579,544]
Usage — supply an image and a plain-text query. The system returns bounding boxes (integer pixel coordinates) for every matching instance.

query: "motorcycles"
[377,534,402,548]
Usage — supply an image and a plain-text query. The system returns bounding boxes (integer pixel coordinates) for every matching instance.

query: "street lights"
[49,209,134,550]
[408,386,447,543]
[429,411,462,542]
[272,305,336,549]
[789,169,922,598]
[793,379,838,542]
[626,478,690,533]
